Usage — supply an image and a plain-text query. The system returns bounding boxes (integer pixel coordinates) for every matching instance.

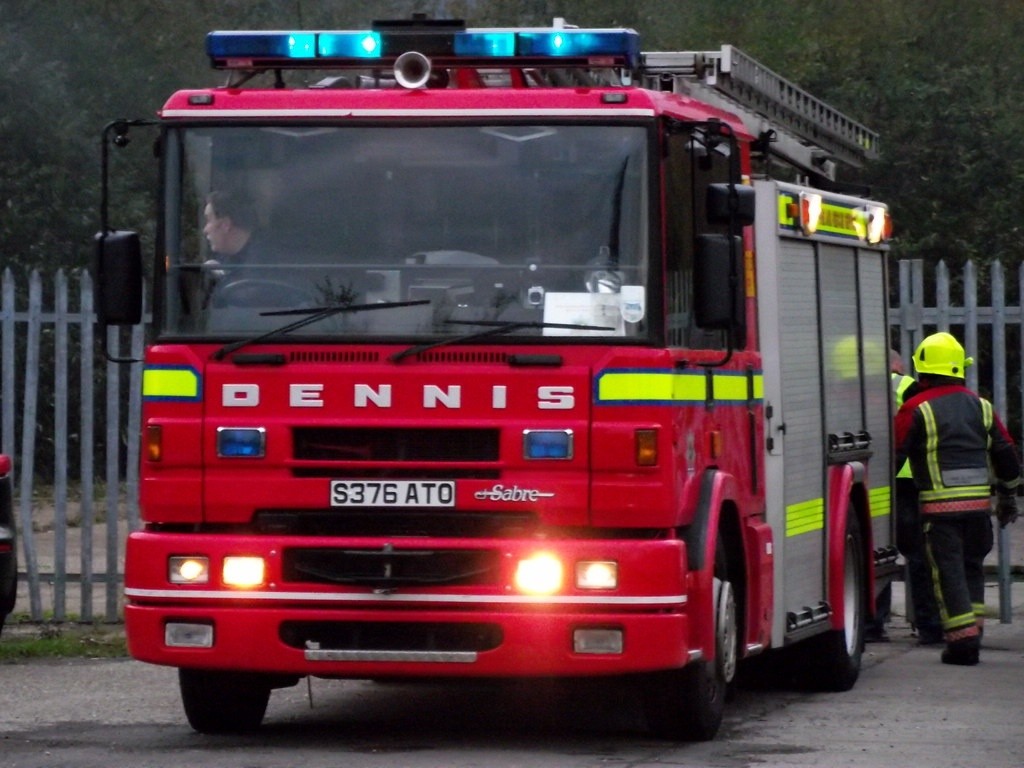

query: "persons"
[864,332,1021,667]
[201,188,278,279]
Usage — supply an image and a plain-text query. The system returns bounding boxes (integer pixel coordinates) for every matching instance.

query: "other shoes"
[919,624,940,642]
[941,640,979,665]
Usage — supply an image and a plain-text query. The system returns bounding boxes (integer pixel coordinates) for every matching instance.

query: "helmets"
[912,331,966,381]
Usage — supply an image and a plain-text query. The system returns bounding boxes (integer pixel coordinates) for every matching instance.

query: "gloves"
[995,496,1018,528]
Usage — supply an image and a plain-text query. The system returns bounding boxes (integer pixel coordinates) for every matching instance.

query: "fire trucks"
[93,15,894,740]
